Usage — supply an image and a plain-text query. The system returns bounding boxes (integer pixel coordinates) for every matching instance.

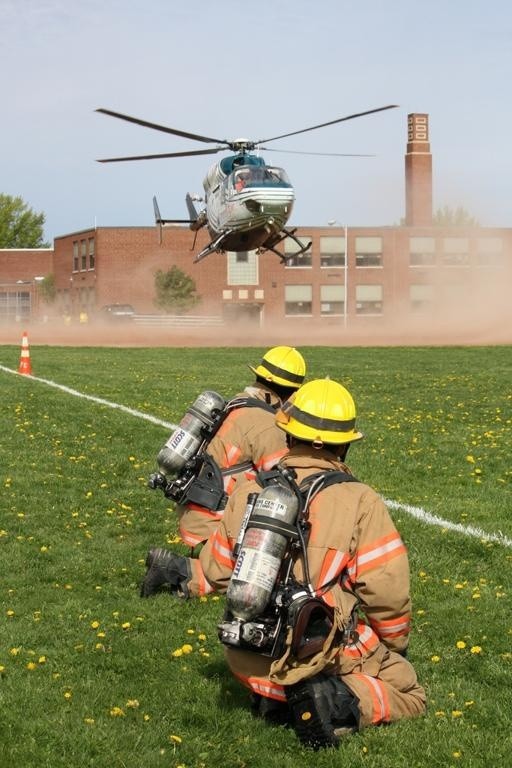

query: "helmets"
[275,379,363,445]
[248,346,306,389]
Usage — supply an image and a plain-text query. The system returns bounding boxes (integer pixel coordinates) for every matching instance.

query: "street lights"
[328,219,348,335]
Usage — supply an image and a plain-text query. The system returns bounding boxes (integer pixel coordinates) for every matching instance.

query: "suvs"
[93,304,136,326]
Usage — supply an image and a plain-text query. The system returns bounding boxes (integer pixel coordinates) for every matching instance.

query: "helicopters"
[93,105,399,264]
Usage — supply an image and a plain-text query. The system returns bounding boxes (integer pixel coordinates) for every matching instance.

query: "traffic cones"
[18,333,32,374]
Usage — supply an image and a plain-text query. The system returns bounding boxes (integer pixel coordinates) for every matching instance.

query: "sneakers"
[284,672,340,750]
[141,548,176,598]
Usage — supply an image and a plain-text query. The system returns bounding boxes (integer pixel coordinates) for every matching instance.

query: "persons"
[140,345,307,603]
[236,175,250,192]
[198,374,426,751]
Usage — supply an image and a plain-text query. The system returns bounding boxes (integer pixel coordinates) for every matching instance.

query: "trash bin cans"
[224,301,264,328]
